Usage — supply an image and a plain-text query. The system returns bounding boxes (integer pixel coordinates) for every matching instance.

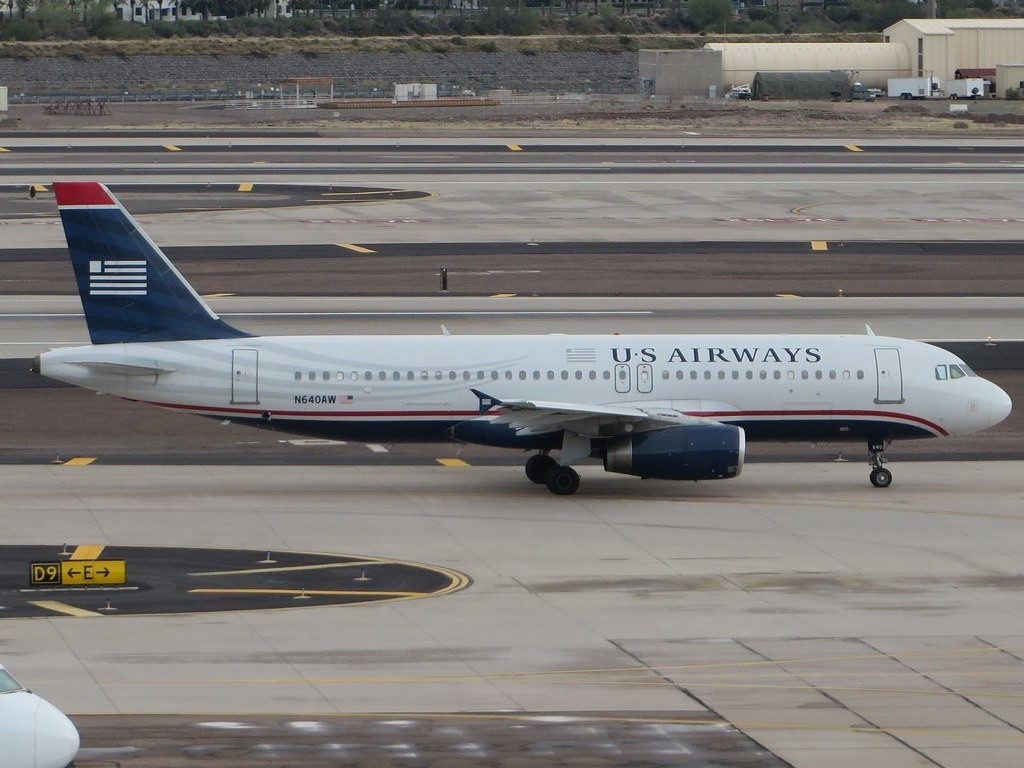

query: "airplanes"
[28,180,1016,498]
